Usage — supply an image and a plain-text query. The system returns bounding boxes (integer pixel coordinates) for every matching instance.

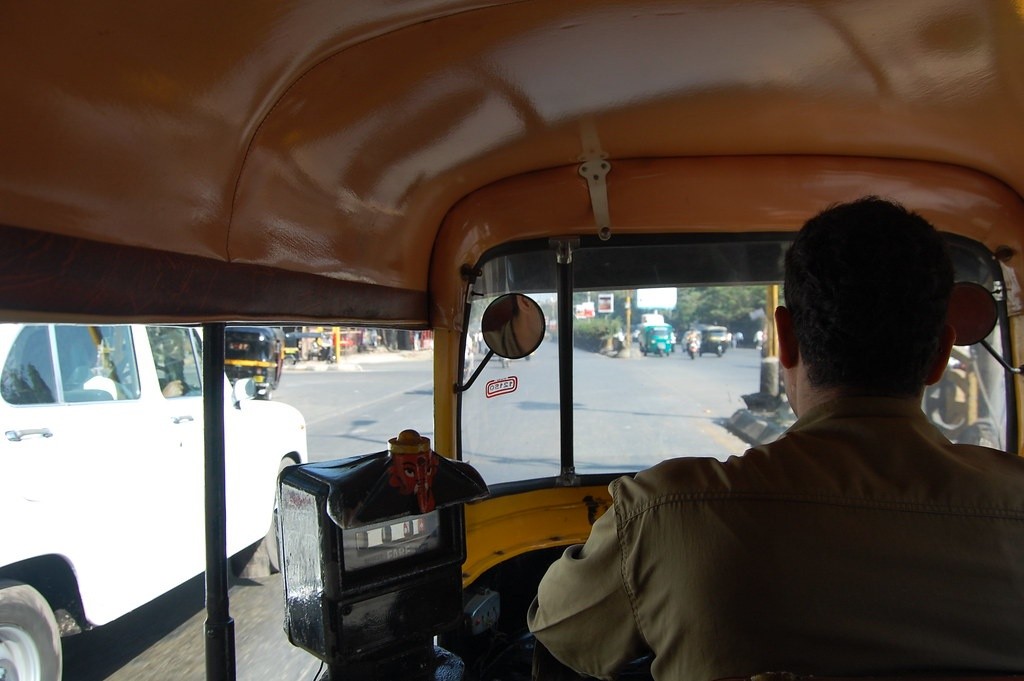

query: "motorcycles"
[286,332,331,365]
[700,325,729,357]
[639,324,673,358]
[223,325,287,401]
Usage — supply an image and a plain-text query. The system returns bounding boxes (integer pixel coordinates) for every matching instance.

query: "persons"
[162,379,185,398]
[526,197,1024,681]
[312,337,320,350]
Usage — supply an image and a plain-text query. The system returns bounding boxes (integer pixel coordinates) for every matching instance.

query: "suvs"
[1,323,311,681]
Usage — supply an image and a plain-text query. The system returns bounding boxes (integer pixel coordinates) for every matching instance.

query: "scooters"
[683,331,701,360]
